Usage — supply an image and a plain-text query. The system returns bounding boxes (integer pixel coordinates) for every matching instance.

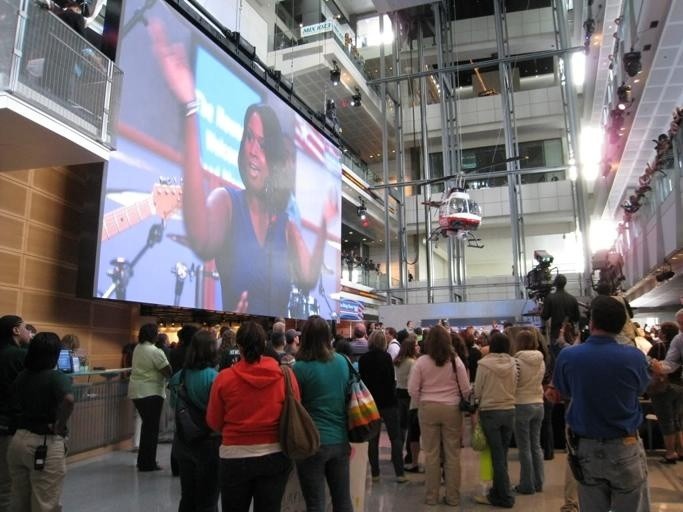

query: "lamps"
[593,50,641,178]
[348,86,362,108]
[327,58,341,86]
[656,258,674,282]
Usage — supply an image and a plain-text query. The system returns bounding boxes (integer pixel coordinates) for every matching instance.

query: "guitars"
[99,176,183,239]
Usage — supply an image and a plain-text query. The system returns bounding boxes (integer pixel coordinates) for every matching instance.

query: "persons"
[5,331,74,511]
[471,184,474,189]
[597,16,682,234]
[483,180,486,187]
[520,176,527,184]
[41,1,107,103]
[147,13,338,315]
[551,296,651,512]
[648,309,682,380]
[477,181,483,188]
[231,289,248,316]
[541,276,579,339]
[341,248,381,283]
[537,175,545,183]
[501,177,507,186]
[407,274,413,282]
[0,315,31,511]
[550,176,559,181]
[585,282,636,347]
[355,153,367,168]
[61,334,80,354]
[25,325,37,341]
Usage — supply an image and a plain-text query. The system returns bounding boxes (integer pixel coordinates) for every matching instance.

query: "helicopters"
[354,152,531,249]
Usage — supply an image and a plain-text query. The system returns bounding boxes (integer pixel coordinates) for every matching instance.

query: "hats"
[284,328,301,339]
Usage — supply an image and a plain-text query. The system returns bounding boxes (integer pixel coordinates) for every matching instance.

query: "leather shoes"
[474,495,492,505]
[370,464,422,483]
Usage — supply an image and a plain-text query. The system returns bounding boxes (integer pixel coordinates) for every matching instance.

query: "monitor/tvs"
[55,349,74,373]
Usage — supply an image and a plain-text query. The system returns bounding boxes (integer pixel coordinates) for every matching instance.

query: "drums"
[290,293,320,319]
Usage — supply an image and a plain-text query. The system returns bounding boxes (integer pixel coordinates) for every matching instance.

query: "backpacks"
[278,366,323,460]
[174,374,213,442]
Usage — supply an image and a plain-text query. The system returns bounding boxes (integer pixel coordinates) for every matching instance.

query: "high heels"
[659,455,679,466]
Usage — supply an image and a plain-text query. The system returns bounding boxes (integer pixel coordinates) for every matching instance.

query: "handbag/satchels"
[644,342,674,398]
[341,352,383,445]
[473,420,494,481]
[460,393,476,413]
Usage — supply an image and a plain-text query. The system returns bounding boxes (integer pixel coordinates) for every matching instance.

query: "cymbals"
[107,189,179,220]
[168,233,189,248]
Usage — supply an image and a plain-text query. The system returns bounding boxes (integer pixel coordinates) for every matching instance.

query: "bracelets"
[177,99,198,119]
